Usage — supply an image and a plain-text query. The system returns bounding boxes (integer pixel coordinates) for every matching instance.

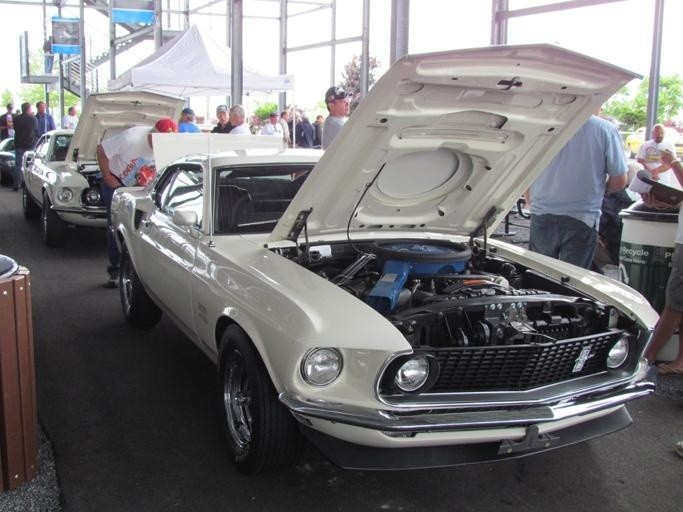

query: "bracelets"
[669,158,681,166]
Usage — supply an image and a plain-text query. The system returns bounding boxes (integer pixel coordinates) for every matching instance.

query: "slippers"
[659,361,683,376]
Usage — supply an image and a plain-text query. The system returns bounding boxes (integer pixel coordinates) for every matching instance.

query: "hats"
[326,87,352,103]
[156,120,177,132]
[216,105,228,113]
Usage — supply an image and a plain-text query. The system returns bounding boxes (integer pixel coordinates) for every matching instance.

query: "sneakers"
[107,273,120,286]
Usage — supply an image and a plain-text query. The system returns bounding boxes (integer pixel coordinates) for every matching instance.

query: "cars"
[108,38,661,480]
[0,139,24,188]
[17,90,187,244]
[602,116,628,137]
[625,121,683,162]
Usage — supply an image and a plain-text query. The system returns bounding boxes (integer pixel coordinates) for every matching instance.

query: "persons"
[211,104,324,148]
[323,85,351,150]
[61,106,79,129]
[96,118,178,286]
[1,101,54,192]
[177,108,200,133]
[635,123,683,208]
[642,148,683,375]
[524,105,629,270]
